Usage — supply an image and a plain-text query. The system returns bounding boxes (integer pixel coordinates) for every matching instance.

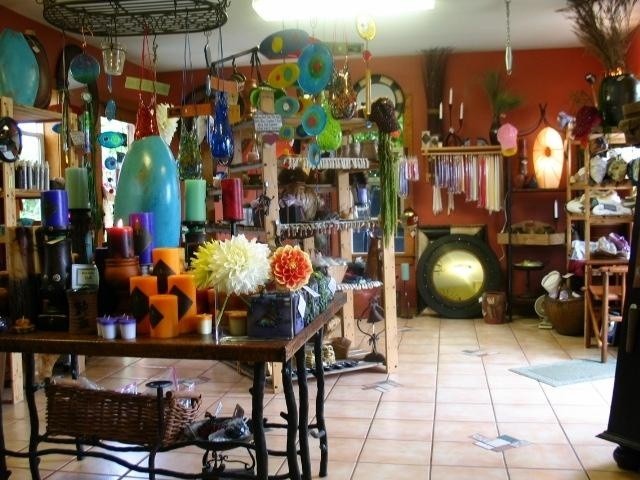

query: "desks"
[3,290,348,480]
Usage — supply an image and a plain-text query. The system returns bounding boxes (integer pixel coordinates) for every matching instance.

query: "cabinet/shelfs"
[505,102,640,364]
[205,74,401,394]
[0,94,106,289]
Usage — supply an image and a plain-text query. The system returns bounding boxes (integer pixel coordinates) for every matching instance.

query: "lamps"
[438,87,464,146]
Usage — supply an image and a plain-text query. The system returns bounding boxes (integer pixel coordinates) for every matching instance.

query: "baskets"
[292,345,337,372]
[43,375,203,450]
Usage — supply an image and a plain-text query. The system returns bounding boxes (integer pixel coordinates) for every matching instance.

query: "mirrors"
[416,233,503,320]
[350,76,404,122]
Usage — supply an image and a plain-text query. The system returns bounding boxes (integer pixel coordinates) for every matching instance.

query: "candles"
[34,162,96,230]
[94,219,247,341]
[184,175,245,222]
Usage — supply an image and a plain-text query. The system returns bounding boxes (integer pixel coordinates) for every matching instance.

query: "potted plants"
[477,67,524,146]
[417,46,447,143]
[555,0,640,127]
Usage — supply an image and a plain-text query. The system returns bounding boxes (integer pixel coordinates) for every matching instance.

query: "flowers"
[190,232,313,296]
